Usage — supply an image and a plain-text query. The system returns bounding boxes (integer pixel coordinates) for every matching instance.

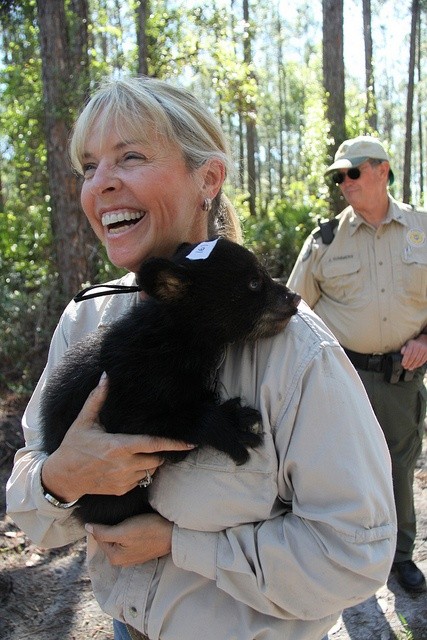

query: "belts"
[339,345,420,372]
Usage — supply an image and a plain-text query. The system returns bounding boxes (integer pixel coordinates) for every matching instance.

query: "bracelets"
[44,493,78,508]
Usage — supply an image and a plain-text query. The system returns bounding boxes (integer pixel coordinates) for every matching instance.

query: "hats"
[324,136,394,185]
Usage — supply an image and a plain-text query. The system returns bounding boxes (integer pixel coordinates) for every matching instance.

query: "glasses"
[332,163,381,185]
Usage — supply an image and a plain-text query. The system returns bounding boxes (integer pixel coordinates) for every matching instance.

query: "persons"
[284,137,427,593]
[5,75,396,639]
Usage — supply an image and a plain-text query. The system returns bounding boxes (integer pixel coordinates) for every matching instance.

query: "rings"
[137,469,152,488]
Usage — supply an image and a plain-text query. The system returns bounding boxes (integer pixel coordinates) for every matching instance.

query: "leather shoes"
[390,559,427,593]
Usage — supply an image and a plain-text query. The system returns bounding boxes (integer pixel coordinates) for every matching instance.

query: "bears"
[35,233,305,528]
